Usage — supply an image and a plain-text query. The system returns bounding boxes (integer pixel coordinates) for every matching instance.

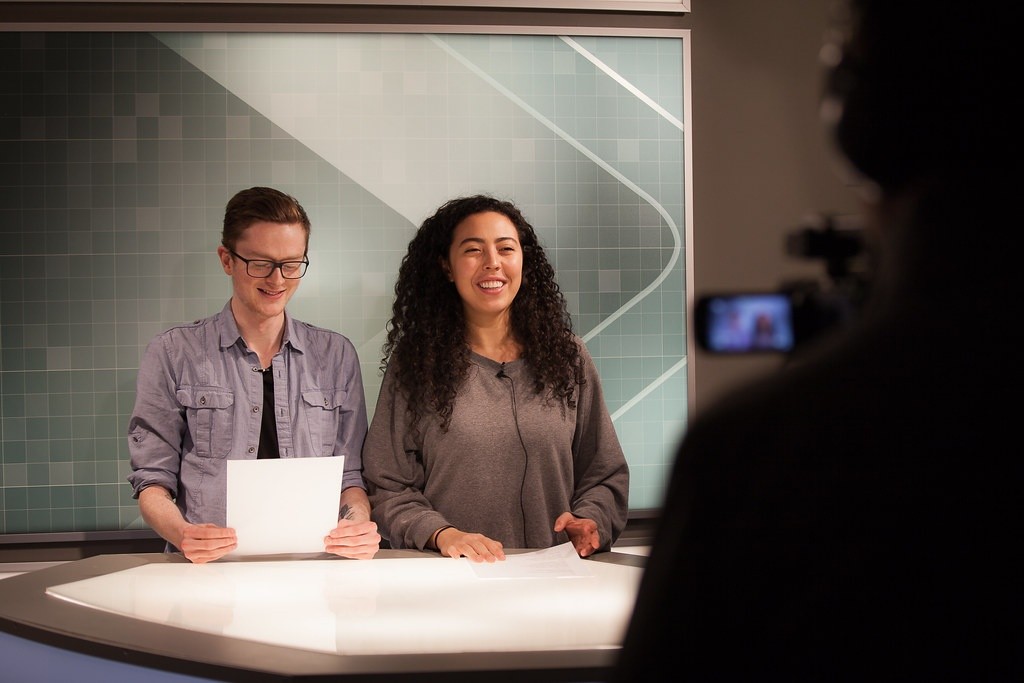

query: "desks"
[0,544,654,682]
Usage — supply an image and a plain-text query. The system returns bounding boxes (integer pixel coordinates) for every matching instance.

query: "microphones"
[496,370,510,378]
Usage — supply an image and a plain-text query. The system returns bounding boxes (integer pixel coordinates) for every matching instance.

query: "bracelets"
[434,525,457,550]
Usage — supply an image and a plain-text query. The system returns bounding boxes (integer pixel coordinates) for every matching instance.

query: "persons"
[362,194,629,563]
[126,187,381,564]
[610,0,1024,682]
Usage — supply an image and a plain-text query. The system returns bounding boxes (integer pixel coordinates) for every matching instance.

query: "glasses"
[233,251,309,280]
